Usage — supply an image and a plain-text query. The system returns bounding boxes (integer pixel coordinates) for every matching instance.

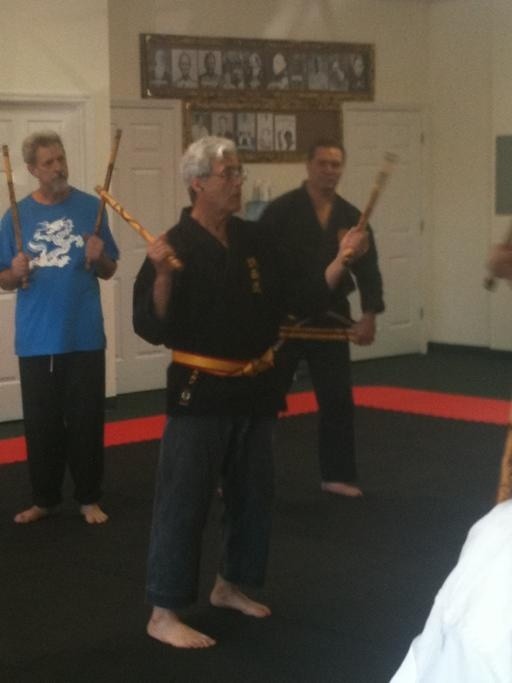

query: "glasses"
[204,167,248,181]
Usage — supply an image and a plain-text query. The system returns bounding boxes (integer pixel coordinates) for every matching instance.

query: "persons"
[146,48,172,88]
[175,52,198,89]
[190,113,209,141]
[267,52,291,91]
[258,138,387,498]
[334,53,368,91]
[0,131,121,525]
[130,134,280,650]
[216,116,234,141]
[245,53,265,90]
[199,52,222,87]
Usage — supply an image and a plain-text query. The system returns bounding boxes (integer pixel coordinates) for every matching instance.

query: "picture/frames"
[139,33,378,103]
[182,99,347,166]
